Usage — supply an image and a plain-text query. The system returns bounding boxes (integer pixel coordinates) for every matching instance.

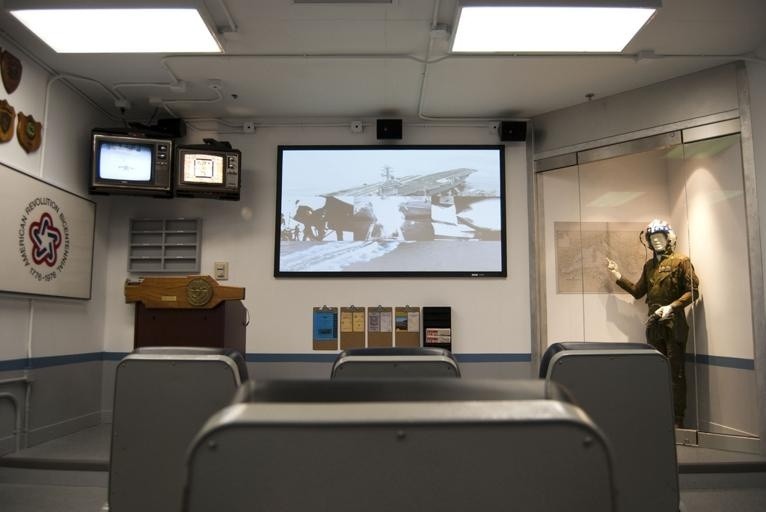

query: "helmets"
[646,220,676,254]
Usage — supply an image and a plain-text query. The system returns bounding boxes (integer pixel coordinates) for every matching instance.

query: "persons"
[293,223,300,240]
[605,218,700,429]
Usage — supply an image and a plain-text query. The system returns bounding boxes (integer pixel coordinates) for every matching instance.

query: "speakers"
[157,118,186,137]
[377,119,402,139]
[499,120,527,141]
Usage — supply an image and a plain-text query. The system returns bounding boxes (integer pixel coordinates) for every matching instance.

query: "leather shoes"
[675,415,683,427]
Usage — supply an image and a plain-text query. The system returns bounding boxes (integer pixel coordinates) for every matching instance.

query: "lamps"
[5,1,235,64]
[444,0,661,69]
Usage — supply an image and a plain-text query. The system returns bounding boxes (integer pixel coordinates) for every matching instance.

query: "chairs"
[108,347,244,512]
[539,342,680,511]
[331,348,459,377]
[186,379,621,511]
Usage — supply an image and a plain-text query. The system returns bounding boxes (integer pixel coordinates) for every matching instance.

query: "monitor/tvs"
[176,147,241,194]
[92,134,172,192]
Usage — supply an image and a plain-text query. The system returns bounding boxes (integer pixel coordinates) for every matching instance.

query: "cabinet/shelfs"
[127,218,201,275]
